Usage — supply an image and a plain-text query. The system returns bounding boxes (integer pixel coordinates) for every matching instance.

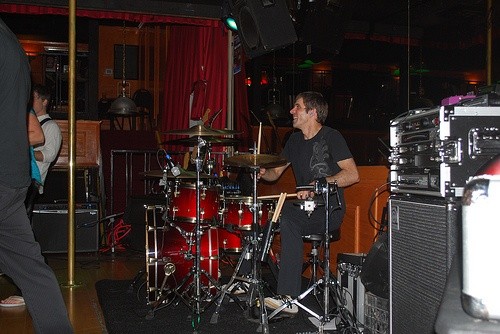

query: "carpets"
[95,278,336,334]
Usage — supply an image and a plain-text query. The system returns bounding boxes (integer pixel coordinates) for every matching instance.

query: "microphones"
[76,223,86,229]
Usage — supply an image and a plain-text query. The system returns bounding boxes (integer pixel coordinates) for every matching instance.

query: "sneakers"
[256,296,298,314]
[221,279,251,294]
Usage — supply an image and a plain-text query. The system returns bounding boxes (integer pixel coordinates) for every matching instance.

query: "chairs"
[129,89,153,130]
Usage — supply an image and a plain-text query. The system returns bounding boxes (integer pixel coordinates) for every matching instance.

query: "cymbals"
[159,127,243,137]
[168,139,242,145]
[222,154,288,170]
[136,169,217,179]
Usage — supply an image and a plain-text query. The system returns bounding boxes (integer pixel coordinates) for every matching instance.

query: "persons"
[0,18,74,334]
[222,91,361,316]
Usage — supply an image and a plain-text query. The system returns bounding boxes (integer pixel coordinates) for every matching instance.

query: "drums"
[220,193,281,234]
[166,182,224,226]
[217,224,248,253]
[142,203,220,305]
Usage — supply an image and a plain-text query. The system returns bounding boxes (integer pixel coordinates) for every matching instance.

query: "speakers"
[230,0,298,59]
[386,193,460,334]
[30,208,98,252]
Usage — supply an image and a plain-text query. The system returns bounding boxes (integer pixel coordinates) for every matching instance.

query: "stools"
[303,230,339,295]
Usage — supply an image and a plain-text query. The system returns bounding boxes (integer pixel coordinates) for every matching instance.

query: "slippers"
[0,295,27,307]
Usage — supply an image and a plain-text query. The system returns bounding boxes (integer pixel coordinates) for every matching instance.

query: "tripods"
[152,137,245,328]
[264,184,361,334]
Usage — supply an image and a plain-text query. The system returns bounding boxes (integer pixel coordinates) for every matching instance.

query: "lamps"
[108,21,140,117]
